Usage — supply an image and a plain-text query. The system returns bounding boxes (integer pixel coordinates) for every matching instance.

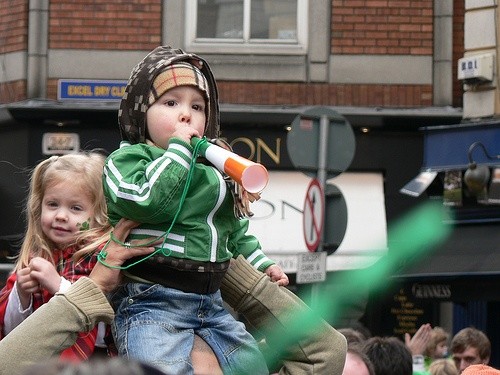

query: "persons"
[0,152,114,365]
[101,45,289,375]
[0,218,347,375]
[336,324,500,375]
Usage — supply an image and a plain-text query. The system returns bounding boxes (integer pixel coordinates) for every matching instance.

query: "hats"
[142,62,211,136]
